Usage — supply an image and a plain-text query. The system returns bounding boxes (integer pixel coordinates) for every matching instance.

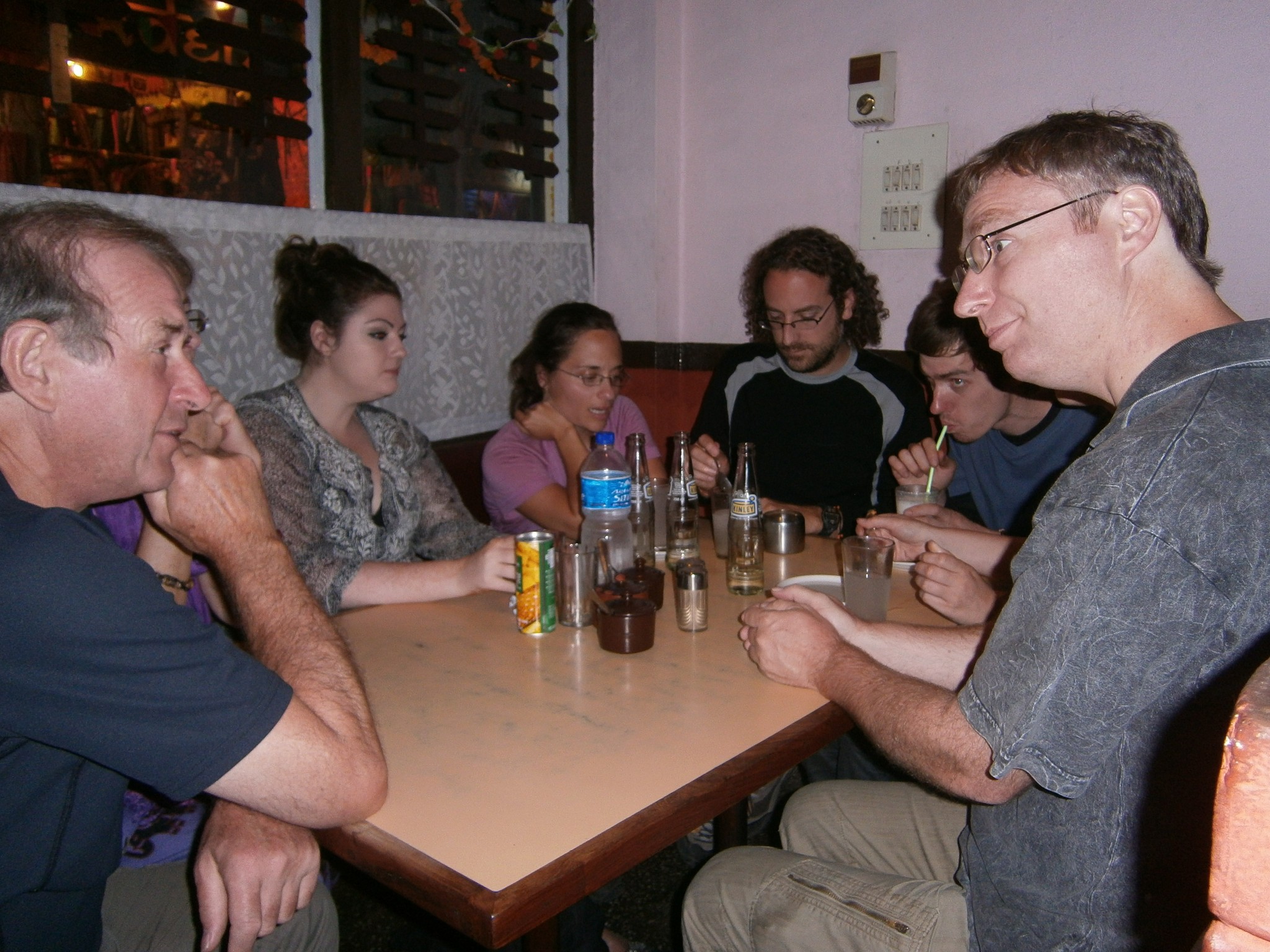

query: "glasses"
[757,294,837,330]
[184,309,211,332]
[556,366,631,388]
[951,190,1118,293]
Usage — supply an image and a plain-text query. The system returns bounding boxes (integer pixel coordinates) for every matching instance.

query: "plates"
[775,574,849,611]
[891,560,924,572]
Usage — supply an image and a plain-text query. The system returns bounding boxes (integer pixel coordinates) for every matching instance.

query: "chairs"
[1192,658,1270,952]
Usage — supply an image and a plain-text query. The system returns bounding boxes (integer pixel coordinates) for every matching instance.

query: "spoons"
[713,456,733,493]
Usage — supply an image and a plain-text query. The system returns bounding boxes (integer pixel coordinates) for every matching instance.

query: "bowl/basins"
[596,590,655,655]
[614,558,666,611]
[762,508,806,555]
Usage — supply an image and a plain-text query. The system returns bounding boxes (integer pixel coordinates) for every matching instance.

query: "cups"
[895,484,941,514]
[555,544,600,627]
[710,484,730,560]
[841,535,897,622]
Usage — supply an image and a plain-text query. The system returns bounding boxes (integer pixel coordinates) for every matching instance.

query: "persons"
[803,278,1111,784]
[682,109,1270,952]
[482,302,667,544]
[0,199,388,952]
[683,227,932,852]
[231,233,515,610]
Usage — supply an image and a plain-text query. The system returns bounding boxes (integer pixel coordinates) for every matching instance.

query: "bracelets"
[154,572,195,592]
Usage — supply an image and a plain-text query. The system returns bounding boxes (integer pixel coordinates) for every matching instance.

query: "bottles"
[579,431,635,586]
[666,433,701,571]
[675,565,710,631]
[622,433,656,568]
[726,442,765,596]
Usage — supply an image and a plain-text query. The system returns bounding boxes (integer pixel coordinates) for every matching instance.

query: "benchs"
[605,336,950,509]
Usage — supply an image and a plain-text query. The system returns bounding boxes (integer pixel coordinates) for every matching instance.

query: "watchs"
[816,501,841,537]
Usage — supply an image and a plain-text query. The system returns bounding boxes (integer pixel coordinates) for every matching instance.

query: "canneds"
[514,531,557,637]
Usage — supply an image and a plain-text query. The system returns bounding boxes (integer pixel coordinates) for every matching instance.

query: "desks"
[260,515,1004,952]
